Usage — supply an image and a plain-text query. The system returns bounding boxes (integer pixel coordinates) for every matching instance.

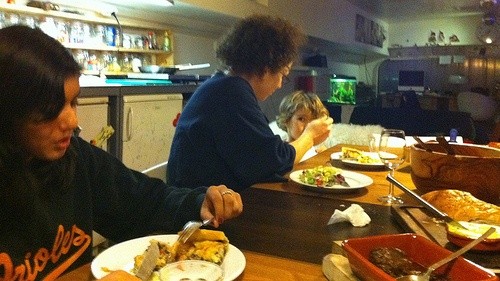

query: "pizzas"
[134,229,229,272]
[341,146,362,160]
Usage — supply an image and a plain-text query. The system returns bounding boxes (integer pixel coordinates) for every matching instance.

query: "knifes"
[136,243,159,280]
[385,176,468,230]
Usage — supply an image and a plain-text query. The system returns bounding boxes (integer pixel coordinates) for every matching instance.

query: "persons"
[166,12,333,194]
[0,23,243,281]
[276,90,329,163]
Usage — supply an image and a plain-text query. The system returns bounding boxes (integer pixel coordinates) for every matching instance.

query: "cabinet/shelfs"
[0,4,174,76]
[73,83,200,188]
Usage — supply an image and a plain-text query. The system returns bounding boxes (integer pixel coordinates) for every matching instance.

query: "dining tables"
[48,145,500,281]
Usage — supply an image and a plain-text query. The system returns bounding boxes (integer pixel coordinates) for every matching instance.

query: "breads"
[420,189,500,226]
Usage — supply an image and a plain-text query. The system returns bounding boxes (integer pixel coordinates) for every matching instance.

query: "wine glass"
[378,129,406,204]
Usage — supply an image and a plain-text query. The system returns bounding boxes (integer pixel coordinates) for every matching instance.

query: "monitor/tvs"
[398,71,424,92]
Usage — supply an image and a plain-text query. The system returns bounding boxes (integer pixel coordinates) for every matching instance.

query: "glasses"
[279,72,290,88]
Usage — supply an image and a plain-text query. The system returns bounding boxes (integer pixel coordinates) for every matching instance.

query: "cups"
[367,126,389,152]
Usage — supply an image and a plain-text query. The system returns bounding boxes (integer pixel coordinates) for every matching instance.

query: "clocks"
[476,21,500,45]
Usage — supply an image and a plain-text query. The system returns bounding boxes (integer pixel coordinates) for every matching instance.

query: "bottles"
[0,9,144,73]
[147,32,154,50]
[163,31,170,51]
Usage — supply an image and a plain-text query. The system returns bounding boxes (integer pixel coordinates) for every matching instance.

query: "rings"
[221,191,232,197]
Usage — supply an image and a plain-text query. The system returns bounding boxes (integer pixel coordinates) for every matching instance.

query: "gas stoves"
[126,72,211,82]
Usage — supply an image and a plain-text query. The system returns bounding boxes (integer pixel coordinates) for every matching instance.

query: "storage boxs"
[327,75,358,104]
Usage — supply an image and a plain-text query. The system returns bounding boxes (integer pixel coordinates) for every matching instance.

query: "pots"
[138,63,211,75]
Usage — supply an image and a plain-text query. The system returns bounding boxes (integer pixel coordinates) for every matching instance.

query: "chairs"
[403,89,476,141]
[456,92,497,141]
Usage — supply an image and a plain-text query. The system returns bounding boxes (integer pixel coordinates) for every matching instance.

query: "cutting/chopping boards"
[390,203,500,276]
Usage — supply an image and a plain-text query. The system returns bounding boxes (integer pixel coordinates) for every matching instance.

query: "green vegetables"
[299,169,316,184]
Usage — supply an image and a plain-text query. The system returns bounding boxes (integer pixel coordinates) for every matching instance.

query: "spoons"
[395,227,496,281]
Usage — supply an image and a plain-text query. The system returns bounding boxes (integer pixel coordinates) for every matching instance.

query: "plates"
[330,152,386,169]
[91,235,245,281]
[289,168,374,191]
[378,151,398,159]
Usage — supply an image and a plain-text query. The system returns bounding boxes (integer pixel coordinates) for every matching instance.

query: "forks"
[177,216,216,243]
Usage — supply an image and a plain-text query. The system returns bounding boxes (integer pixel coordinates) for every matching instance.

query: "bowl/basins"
[159,259,223,281]
[341,232,500,281]
[444,222,500,252]
[410,142,500,205]
